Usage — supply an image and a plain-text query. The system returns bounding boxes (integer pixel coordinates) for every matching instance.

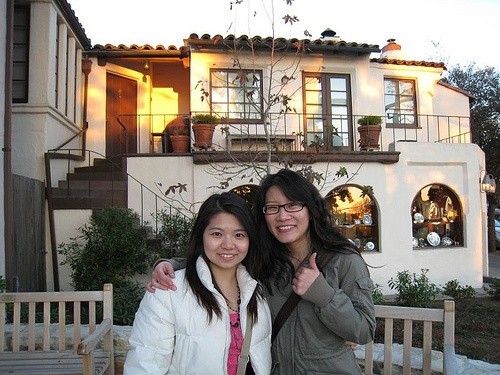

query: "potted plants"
[167,118,189,153]
[191,114,218,148]
[357,115,383,149]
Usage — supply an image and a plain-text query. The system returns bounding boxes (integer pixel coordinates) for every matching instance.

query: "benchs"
[354,300,457,375]
[225,134,296,152]
[0,284,115,375]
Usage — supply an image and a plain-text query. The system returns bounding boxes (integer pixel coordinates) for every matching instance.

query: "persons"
[122,192,276,375]
[146,168,376,375]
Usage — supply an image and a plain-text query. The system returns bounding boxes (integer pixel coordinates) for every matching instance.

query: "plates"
[442,237,453,245]
[427,232,441,247]
[414,212,424,223]
[413,236,418,248]
[366,242,375,250]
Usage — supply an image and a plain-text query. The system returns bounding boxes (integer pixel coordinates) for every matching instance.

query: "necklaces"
[212,278,240,328]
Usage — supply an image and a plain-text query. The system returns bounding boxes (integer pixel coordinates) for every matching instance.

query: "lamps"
[479,170,496,194]
[143,60,149,69]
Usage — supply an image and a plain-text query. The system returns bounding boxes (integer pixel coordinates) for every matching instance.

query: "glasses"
[262,202,306,215]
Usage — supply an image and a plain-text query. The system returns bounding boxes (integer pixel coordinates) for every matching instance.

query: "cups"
[354,219,361,225]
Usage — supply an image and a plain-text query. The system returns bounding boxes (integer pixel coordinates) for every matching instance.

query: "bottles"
[362,213,373,225]
